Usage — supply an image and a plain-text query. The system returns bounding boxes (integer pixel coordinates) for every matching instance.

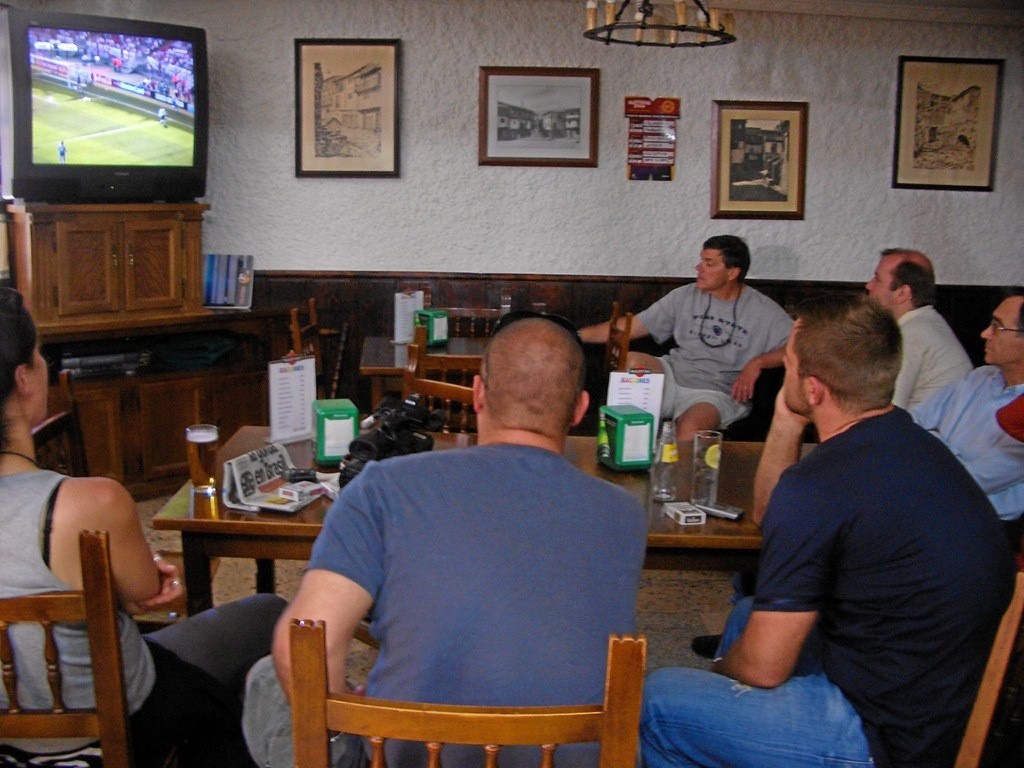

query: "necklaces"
[699,289,749,350]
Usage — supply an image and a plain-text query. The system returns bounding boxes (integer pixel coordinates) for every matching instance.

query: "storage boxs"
[279,481,324,502]
[663,502,706,525]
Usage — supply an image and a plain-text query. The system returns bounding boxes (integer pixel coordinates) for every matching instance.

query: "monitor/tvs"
[0,10,208,206]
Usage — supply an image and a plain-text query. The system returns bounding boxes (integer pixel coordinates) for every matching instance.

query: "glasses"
[484,311,581,388]
[990,318,1024,335]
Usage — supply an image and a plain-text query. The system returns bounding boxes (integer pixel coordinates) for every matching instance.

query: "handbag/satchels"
[242,653,366,768]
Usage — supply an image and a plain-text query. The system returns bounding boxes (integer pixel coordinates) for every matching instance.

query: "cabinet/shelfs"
[45,302,327,501]
[4,203,214,334]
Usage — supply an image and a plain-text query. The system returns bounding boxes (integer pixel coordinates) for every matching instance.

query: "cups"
[691,429,724,507]
[186,424,220,495]
[192,489,218,522]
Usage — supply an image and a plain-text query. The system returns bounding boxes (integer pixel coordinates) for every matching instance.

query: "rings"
[168,579,184,586]
[744,385,749,393]
[154,555,164,560]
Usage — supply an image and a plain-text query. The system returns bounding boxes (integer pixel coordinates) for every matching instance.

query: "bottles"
[651,422,679,501]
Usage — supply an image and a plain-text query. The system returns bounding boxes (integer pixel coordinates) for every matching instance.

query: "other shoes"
[691,634,723,658]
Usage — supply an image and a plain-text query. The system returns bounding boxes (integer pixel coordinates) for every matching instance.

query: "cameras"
[338,394,449,487]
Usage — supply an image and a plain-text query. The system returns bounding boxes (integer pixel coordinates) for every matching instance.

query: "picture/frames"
[479,66,600,168]
[709,100,809,220]
[892,54,1006,191]
[295,37,400,177]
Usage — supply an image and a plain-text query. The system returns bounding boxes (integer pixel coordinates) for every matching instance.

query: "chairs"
[0,290,1024,768]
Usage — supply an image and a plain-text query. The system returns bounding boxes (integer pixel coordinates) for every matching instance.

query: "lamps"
[583,0,737,48]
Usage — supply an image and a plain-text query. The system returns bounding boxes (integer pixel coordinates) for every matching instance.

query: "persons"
[58,140,68,164]
[635,293,1017,768]
[31,30,194,110]
[578,234,795,444]
[158,105,168,128]
[242,316,649,768]
[1,288,287,722]
[864,248,973,407]
[910,293,1024,555]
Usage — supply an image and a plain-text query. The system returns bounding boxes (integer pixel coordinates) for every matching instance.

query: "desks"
[361,336,487,413]
[154,427,820,616]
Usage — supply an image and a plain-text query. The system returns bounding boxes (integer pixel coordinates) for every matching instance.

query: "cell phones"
[695,500,745,522]
